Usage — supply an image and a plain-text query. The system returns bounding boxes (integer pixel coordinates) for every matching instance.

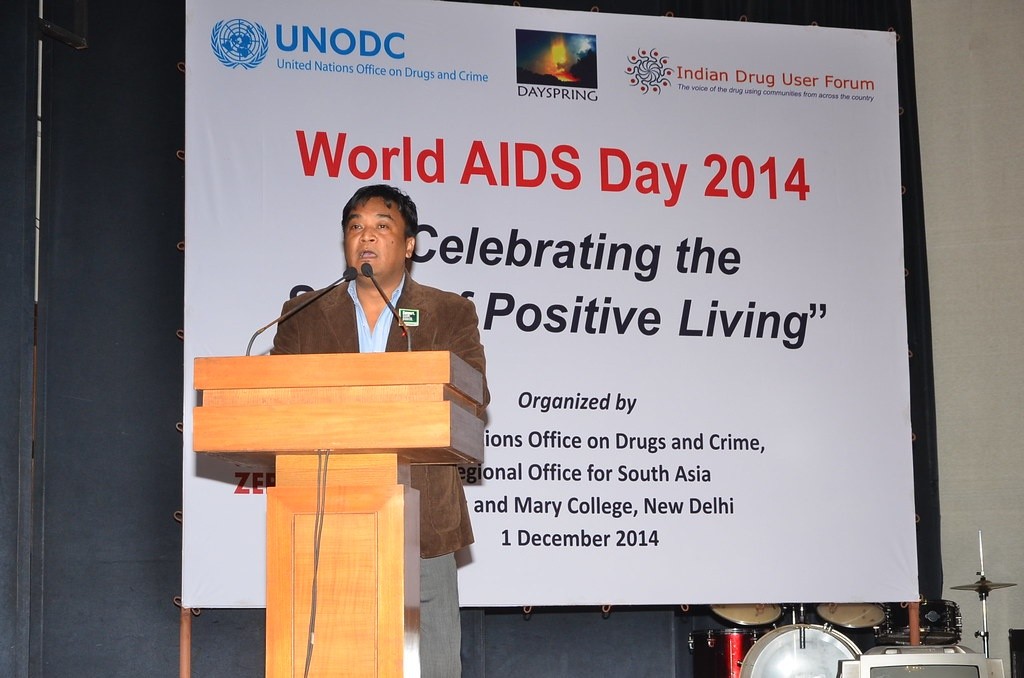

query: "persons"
[268,184,491,678]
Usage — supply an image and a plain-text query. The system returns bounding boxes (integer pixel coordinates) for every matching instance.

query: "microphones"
[360,262,412,352]
[246,267,358,356]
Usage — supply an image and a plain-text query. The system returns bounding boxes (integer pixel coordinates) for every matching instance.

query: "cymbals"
[949,576,1018,598]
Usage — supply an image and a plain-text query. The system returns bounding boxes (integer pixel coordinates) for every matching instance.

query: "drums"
[709,603,788,625]
[873,597,963,646]
[815,602,891,629]
[736,622,863,678]
[688,626,771,678]
